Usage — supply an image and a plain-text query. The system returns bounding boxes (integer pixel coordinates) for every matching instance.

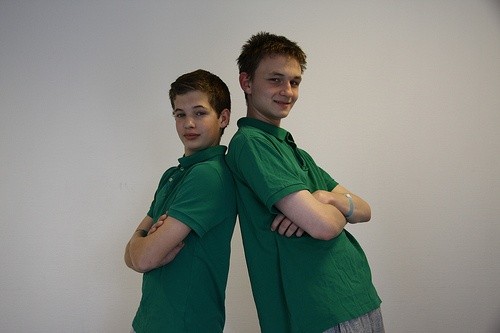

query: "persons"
[221,32,385,332]
[125,67,235,332]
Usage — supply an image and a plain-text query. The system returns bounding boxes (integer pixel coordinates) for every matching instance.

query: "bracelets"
[340,193,353,219]
